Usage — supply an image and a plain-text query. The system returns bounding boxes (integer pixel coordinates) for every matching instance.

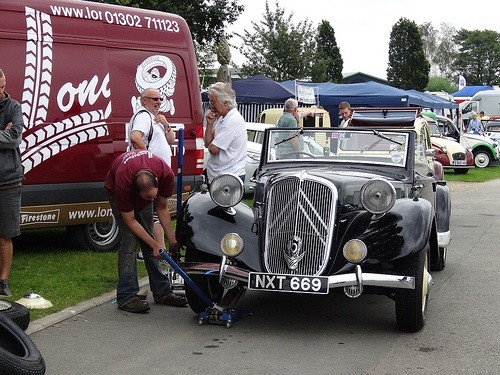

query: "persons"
[128,87,184,263]
[453,111,466,131]
[274,98,316,160]
[104,149,188,313]
[337,102,353,127]
[205,81,248,186]
[0,69,25,294]
[467,111,500,135]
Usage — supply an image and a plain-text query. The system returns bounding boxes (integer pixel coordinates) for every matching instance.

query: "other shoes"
[138,252,144,259]
[121,298,150,313]
[156,294,188,306]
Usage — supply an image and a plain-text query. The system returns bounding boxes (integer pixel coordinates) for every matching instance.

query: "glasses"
[142,97,164,101]
[209,101,218,104]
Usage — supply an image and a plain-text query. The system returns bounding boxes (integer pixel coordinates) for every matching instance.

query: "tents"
[202,73,460,133]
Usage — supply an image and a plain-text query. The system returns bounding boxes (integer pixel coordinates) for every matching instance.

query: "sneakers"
[0,280,10,297]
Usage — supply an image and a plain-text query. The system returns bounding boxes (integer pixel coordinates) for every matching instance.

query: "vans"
[255,107,330,153]
[0,1,204,251]
[457,90,500,130]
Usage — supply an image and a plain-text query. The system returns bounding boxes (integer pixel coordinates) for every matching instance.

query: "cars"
[203,122,336,198]
[420,112,499,169]
[337,107,445,183]
[487,115,499,120]
[480,119,500,158]
[173,126,452,334]
[422,116,476,176]
